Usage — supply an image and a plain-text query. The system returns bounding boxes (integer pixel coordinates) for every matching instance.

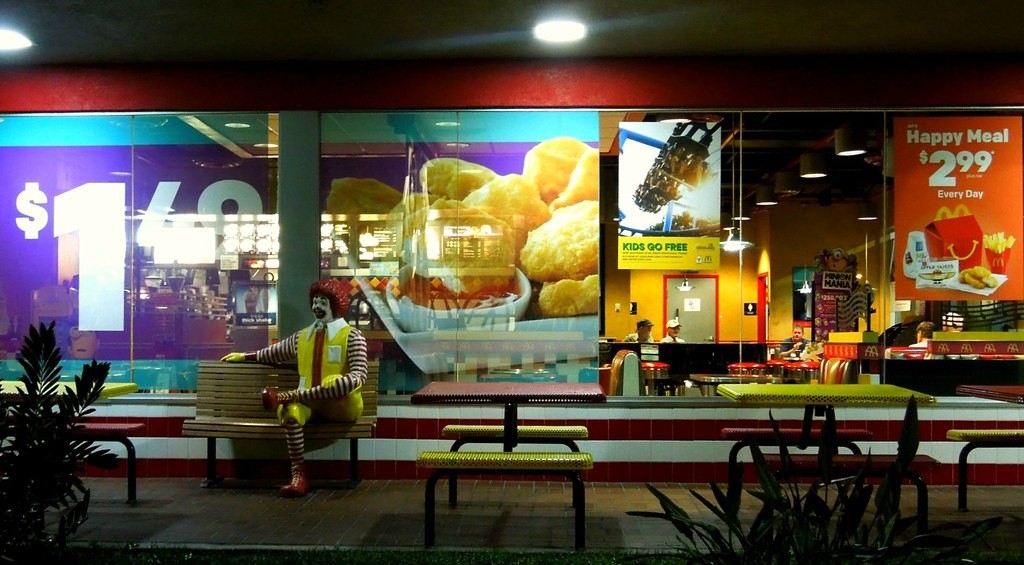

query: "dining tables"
[716,383,936,454]
[410,381,607,452]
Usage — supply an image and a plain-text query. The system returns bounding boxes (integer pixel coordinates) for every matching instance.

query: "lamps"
[774,172,800,193]
[800,153,828,178]
[723,217,738,230]
[835,128,867,156]
[731,207,750,220]
[756,184,778,205]
[795,281,812,294]
[719,133,756,252]
[675,277,695,292]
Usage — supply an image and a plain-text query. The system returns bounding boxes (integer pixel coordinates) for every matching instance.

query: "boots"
[280,472,308,497]
[262,391,299,412]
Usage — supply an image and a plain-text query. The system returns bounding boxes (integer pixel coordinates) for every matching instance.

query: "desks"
[0,380,138,403]
[690,373,783,397]
[956,385,1024,404]
[599,343,767,373]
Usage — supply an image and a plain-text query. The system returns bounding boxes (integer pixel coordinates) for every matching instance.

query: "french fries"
[983,232,1015,254]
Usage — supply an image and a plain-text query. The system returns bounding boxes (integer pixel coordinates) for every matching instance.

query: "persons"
[909,321,939,348]
[660,319,686,343]
[220,280,368,498]
[942,311,964,332]
[779,325,811,359]
[622,319,655,343]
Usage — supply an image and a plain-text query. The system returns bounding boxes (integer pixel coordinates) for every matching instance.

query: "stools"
[641,361,670,396]
[805,362,820,384]
[767,360,789,375]
[752,361,766,374]
[785,362,808,384]
[727,363,754,375]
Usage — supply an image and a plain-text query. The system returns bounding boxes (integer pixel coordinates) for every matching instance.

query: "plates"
[324,211,599,374]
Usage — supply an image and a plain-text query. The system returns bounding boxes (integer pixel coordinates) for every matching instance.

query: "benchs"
[6,422,145,504]
[818,357,858,384]
[608,349,647,396]
[945,429,1024,510]
[760,454,941,534]
[182,360,381,490]
[440,425,589,507]
[417,452,593,549]
[719,428,874,497]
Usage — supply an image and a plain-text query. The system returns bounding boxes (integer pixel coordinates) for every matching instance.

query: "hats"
[637,320,656,331]
[308,279,349,317]
[666,320,682,329]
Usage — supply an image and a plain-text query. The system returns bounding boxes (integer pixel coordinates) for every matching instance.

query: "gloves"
[321,374,342,388]
[222,353,245,361]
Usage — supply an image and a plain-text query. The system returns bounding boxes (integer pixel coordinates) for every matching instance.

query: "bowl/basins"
[385,263,530,333]
